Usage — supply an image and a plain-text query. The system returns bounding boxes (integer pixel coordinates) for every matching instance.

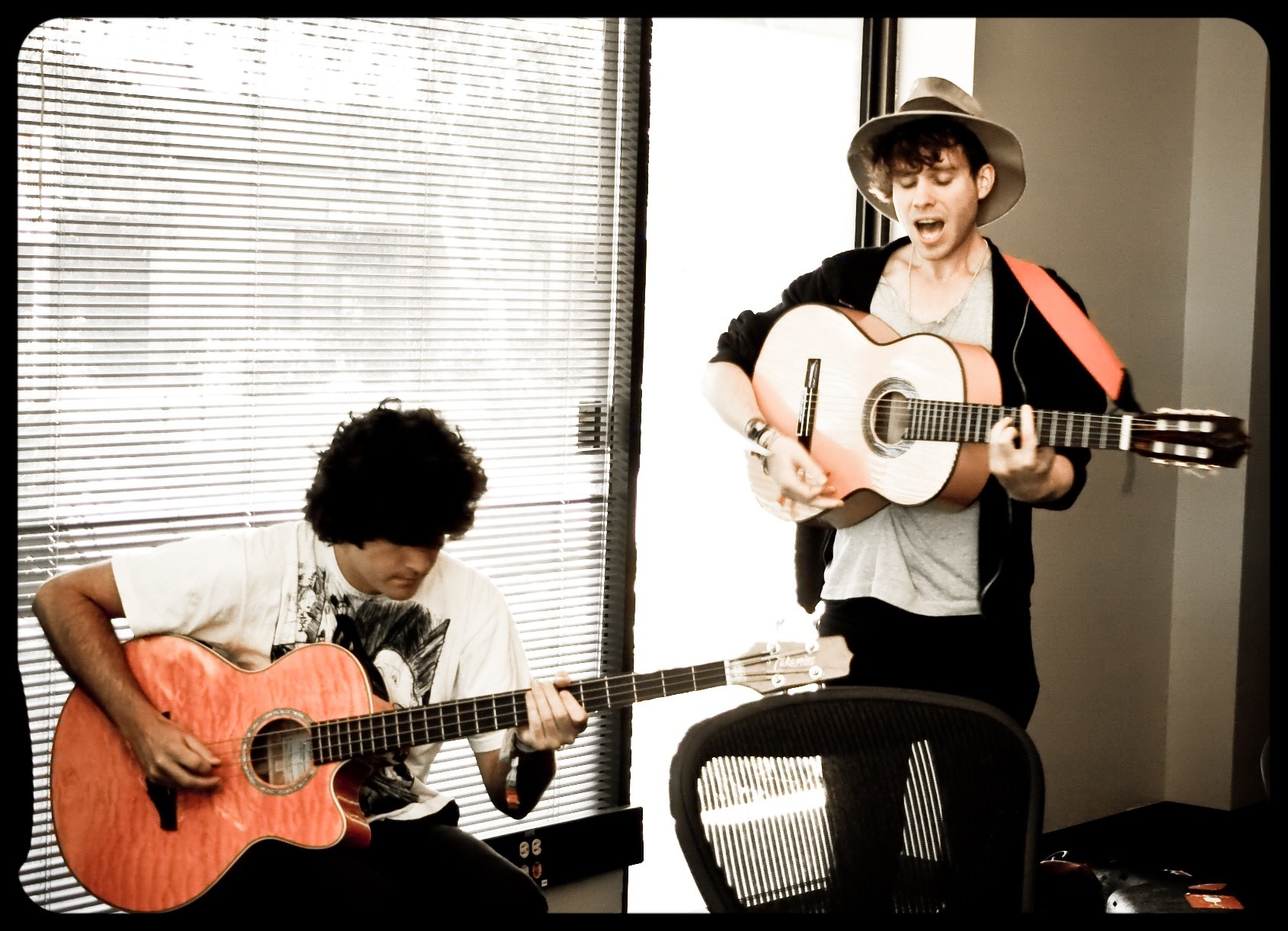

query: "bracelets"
[741,418,781,475]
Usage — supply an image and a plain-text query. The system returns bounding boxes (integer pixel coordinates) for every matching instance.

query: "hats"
[847,77,1027,229]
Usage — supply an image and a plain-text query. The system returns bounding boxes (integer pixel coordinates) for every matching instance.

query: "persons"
[703,77,1107,913]
[33,397,588,913]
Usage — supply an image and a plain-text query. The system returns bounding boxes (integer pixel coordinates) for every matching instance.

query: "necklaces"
[908,239,988,336]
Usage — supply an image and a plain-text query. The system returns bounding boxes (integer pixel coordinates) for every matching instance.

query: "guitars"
[50,629,857,916]
[748,302,1253,530]
